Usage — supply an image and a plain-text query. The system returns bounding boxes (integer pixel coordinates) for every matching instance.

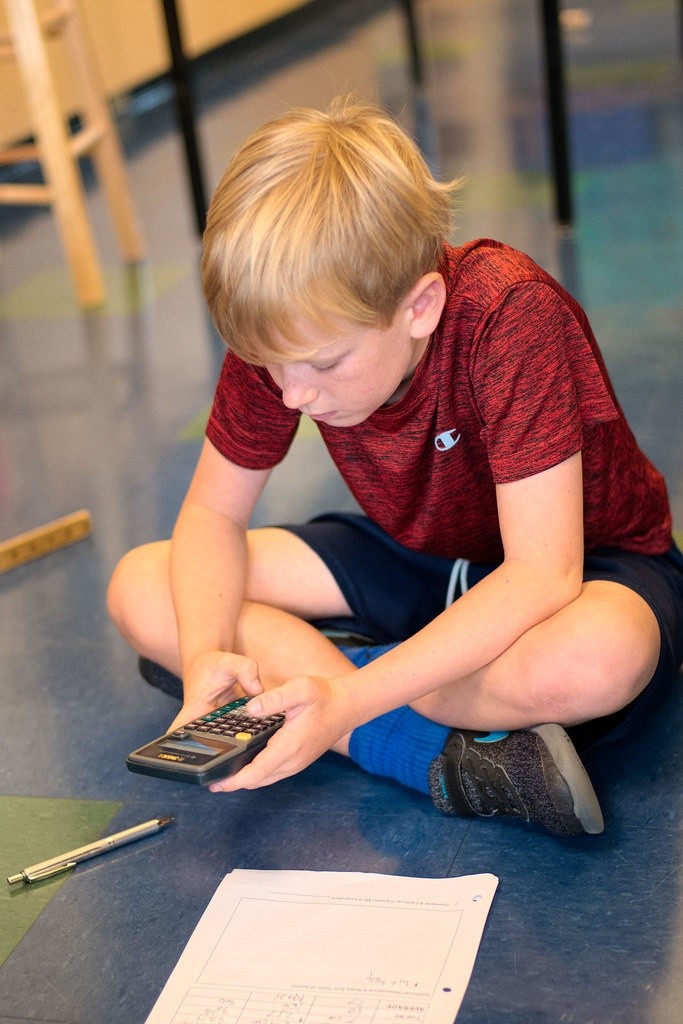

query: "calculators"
[128,694,290,787]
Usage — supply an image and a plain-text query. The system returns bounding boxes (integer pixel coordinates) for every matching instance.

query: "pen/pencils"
[5,807,176,891]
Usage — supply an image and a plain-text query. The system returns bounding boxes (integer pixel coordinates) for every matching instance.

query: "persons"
[108,93,683,835]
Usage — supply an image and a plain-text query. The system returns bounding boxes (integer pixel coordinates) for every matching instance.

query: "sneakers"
[428,723,604,838]
[138,655,184,701]
